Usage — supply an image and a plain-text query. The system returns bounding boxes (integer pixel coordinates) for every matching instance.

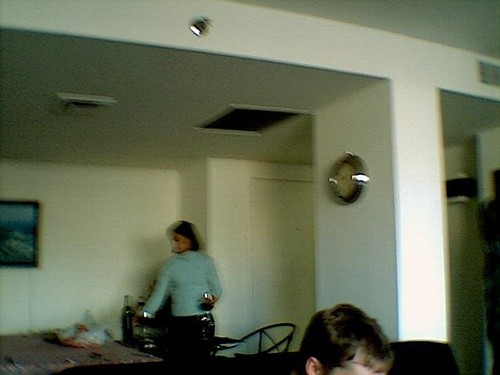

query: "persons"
[299,304,395,375]
[135,221,222,361]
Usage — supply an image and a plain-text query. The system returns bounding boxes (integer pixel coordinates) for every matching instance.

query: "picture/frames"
[0,199,40,267]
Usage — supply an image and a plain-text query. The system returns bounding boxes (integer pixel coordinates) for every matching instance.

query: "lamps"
[190,16,210,37]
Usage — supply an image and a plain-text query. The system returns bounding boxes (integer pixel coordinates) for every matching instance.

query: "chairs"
[214,322,295,356]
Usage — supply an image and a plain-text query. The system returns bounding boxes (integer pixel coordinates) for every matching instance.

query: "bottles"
[119,295,135,342]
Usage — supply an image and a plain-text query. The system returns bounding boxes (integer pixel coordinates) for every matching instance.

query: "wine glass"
[199,290,216,326]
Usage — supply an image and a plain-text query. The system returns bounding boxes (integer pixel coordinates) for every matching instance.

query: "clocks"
[328,153,370,204]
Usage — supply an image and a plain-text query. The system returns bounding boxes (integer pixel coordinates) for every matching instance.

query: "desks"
[1,333,164,375]
